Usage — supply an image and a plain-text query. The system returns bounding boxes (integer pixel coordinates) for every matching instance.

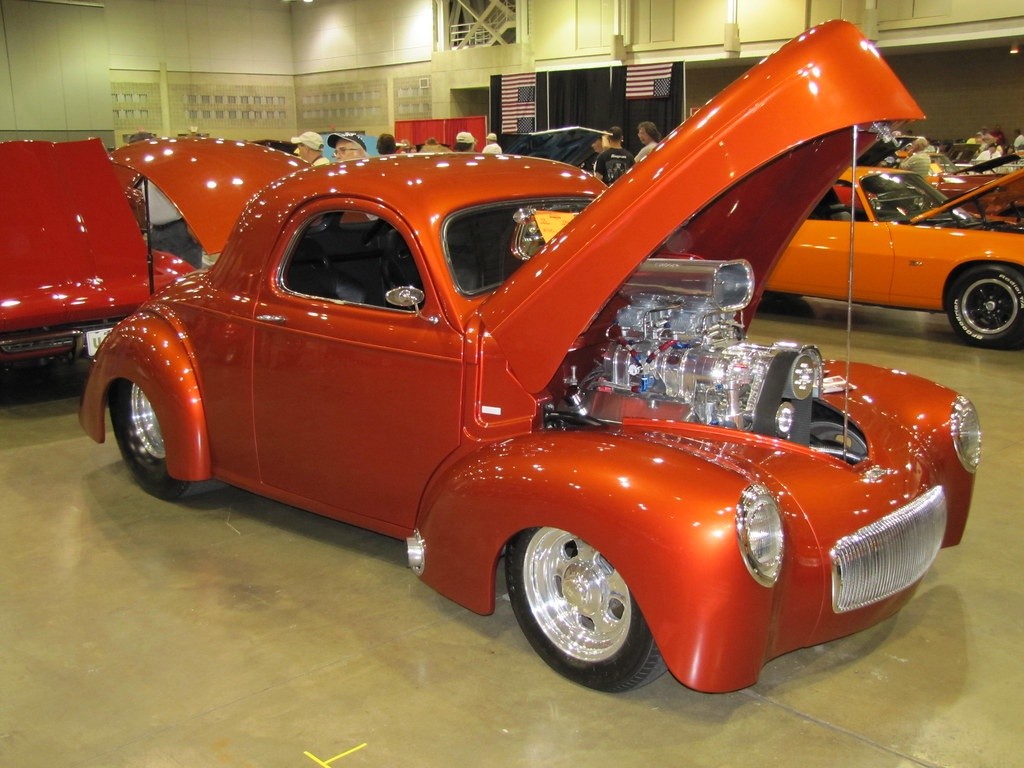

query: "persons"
[899,136,930,180]
[129,130,202,271]
[326,132,379,224]
[453,131,476,152]
[591,126,636,188]
[965,123,1024,160]
[481,133,502,154]
[633,121,662,162]
[377,133,396,155]
[290,132,329,167]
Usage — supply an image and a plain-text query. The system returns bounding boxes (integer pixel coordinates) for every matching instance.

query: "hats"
[976,131,983,136]
[326,133,366,151]
[486,133,497,141]
[456,131,477,144]
[988,142,996,148]
[291,131,324,151]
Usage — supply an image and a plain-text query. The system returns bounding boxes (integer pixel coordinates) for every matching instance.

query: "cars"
[79,13,982,695]
[489,124,1023,352]
[0,135,199,369]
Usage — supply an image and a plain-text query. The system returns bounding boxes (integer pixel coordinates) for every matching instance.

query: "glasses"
[333,147,360,156]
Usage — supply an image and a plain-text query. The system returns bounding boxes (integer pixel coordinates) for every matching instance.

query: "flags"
[502,104,537,133]
[624,63,673,99]
[501,74,537,103]
